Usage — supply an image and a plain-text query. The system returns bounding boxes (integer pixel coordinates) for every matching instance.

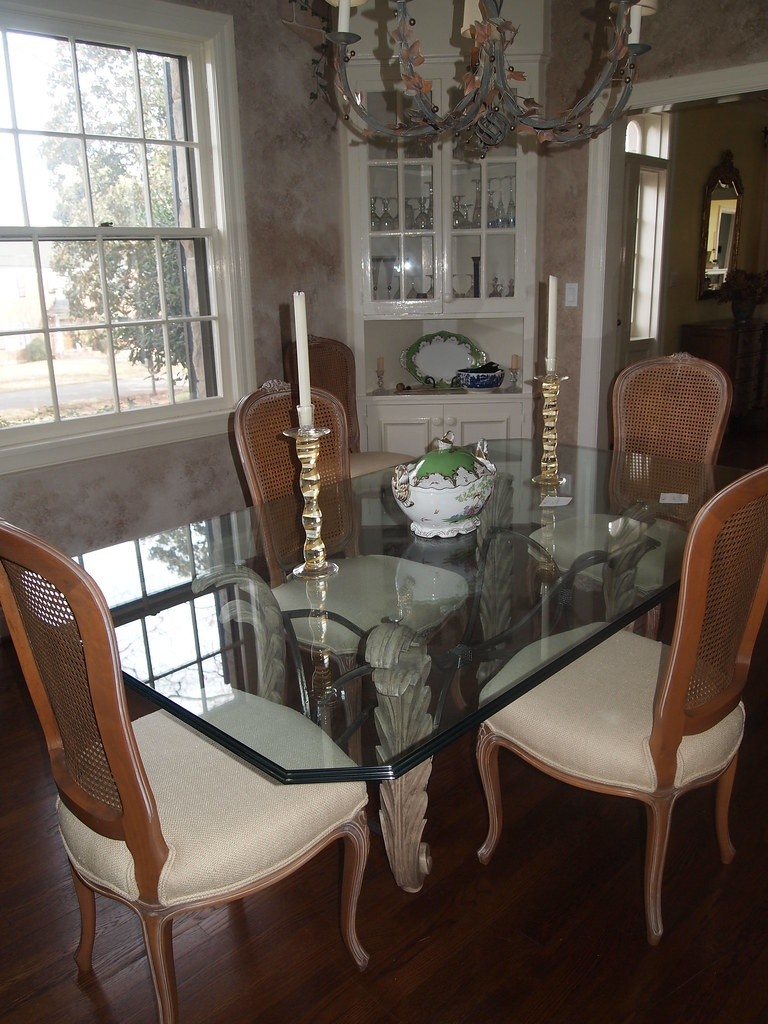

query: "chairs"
[284,333,417,555]
[611,351,733,465]
[0,516,370,1024]
[233,381,470,766]
[476,463,768,958]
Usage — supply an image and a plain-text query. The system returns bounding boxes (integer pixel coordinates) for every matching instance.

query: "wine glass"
[372,256,434,300]
[371,180,433,231]
[452,174,514,230]
[452,273,513,298]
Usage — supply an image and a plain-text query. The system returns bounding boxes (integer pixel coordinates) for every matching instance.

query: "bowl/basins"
[457,368,506,395]
[390,434,497,538]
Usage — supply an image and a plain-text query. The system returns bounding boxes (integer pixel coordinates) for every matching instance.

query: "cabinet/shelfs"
[339,55,551,535]
[680,318,768,440]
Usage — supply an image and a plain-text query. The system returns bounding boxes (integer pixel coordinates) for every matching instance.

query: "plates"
[525,516,691,592]
[268,551,468,655]
[400,331,486,390]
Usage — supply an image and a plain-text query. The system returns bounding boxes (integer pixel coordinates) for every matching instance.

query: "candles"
[547,274,557,373]
[511,354,519,369]
[292,289,313,427]
[376,355,384,370]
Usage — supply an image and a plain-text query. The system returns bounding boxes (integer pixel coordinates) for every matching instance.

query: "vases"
[731,299,756,323]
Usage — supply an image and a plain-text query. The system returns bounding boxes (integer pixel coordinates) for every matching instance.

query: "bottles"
[472,256,480,298]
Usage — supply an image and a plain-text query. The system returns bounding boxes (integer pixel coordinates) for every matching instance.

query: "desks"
[21,437,750,895]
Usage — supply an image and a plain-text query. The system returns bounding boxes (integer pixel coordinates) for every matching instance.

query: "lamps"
[324,0,659,160]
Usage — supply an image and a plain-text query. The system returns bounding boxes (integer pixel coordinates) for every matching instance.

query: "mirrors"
[696,148,745,301]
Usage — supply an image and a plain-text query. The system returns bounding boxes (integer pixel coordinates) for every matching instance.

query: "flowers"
[714,265,768,305]
